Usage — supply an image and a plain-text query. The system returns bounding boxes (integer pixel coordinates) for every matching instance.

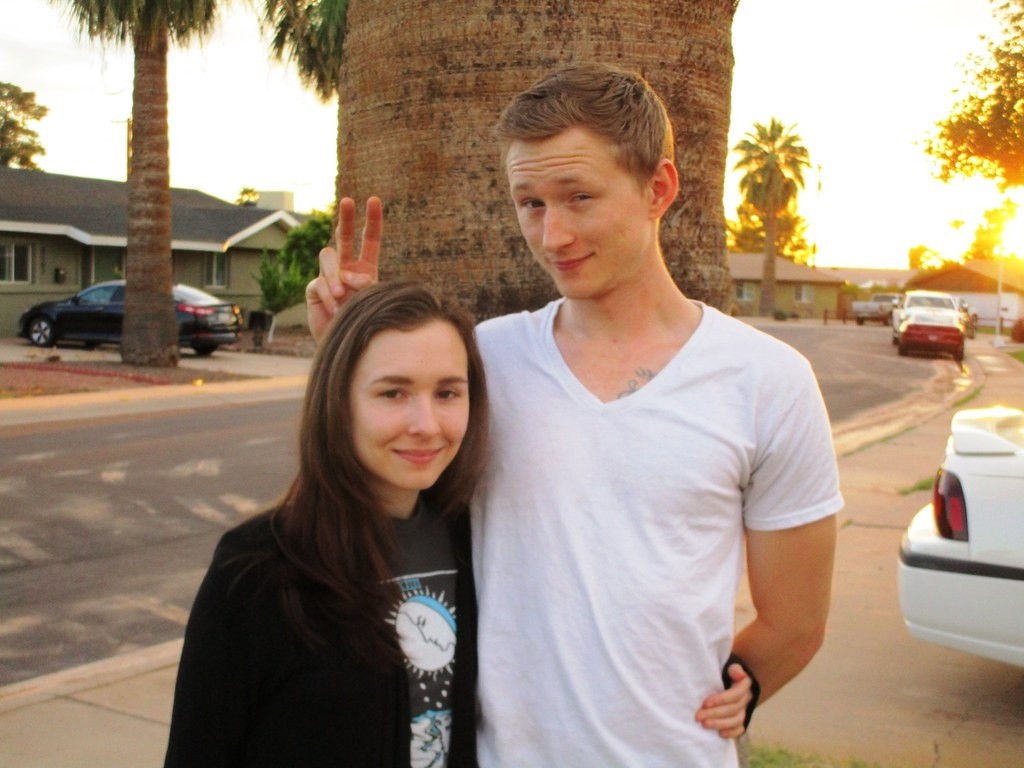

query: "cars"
[897,307,965,361]
[15,279,243,357]
[895,407,1024,661]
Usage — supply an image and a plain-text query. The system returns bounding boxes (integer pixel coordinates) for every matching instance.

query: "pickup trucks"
[850,292,903,328]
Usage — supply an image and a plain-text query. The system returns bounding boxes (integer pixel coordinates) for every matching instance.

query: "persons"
[300,62,847,768]
[163,277,763,767]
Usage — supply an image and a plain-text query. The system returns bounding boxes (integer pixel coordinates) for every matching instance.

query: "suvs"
[892,291,960,347]
